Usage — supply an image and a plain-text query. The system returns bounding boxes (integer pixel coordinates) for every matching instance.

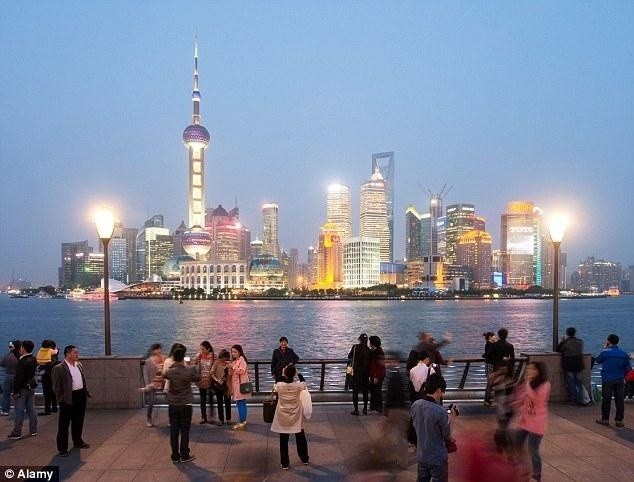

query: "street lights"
[546,210,569,352]
[92,206,116,357]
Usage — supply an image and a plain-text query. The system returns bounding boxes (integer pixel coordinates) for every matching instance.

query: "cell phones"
[604,341,609,347]
[8,341,13,347]
[184,356,191,362]
[432,363,438,368]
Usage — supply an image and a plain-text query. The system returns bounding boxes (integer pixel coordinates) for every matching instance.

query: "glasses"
[7,346,14,349]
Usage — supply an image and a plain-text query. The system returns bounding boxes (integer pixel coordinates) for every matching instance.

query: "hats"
[359,333,367,342]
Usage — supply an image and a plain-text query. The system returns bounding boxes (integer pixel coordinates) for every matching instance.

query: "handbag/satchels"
[208,379,227,395]
[419,366,431,396]
[238,361,252,393]
[262,388,278,424]
[25,357,39,393]
[445,436,457,453]
[345,345,356,376]
[592,385,602,404]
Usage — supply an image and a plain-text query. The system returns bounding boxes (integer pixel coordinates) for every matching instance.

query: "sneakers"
[8,436,21,440]
[172,453,181,464]
[303,455,310,465]
[0,411,8,415]
[146,422,153,427]
[168,424,171,427]
[180,454,195,464]
[280,465,290,471]
[32,432,37,436]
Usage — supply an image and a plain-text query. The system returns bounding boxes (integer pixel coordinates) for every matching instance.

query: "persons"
[7,340,38,440]
[51,345,91,458]
[407,350,436,444]
[624,366,634,399]
[39,341,58,416]
[161,342,188,427]
[482,328,515,395]
[416,330,452,406]
[506,361,552,482]
[142,343,163,427]
[225,344,253,431]
[210,348,232,426]
[558,327,585,407]
[193,340,218,425]
[36,340,59,366]
[271,336,300,383]
[135,370,165,393]
[163,349,202,464]
[270,365,313,470]
[367,335,385,414]
[409,373,459,482]
[593,334,631,427]
[348,333,370,416]
[0,339,21,417]
[482,332,497,407]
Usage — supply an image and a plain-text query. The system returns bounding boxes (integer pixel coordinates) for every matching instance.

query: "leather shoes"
[217,421,225,427]
[59,449,69,457]
[73,442,90,449]
[231,424,246,430]
[226,421,231,426]
[351,411,360,417]
[199,418,208,425]
[52,409,56,411]
[208,416,215,425]
[44,411,50,414]
[243,420,247,426]
[363,408,368,415]
[596,417,609,425]
[616,420,624,426]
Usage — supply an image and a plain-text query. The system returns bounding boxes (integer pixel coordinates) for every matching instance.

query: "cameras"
[445,404,457,413]
